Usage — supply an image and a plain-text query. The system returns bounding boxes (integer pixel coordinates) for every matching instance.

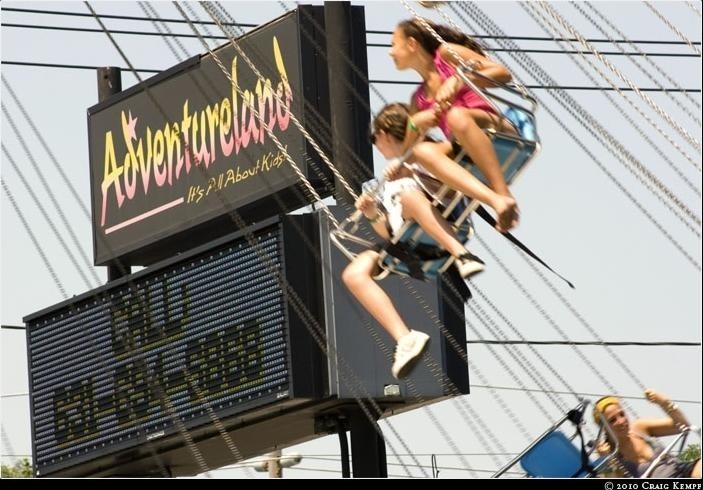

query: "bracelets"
[365,208,384,223]
[407,118,420,135]
[661,399,679,414]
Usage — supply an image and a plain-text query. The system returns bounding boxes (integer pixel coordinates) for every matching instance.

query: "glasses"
[369,134,376,143]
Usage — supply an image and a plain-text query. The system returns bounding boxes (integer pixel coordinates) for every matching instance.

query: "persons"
[339,96,483,382]
[384,17,521,233]
[590,388,703,479]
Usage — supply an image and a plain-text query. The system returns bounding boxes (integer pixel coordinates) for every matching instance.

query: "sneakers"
[457,254,482,279]
[391,329,432,379]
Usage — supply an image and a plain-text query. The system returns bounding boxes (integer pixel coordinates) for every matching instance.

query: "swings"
[4,1,702,480]
